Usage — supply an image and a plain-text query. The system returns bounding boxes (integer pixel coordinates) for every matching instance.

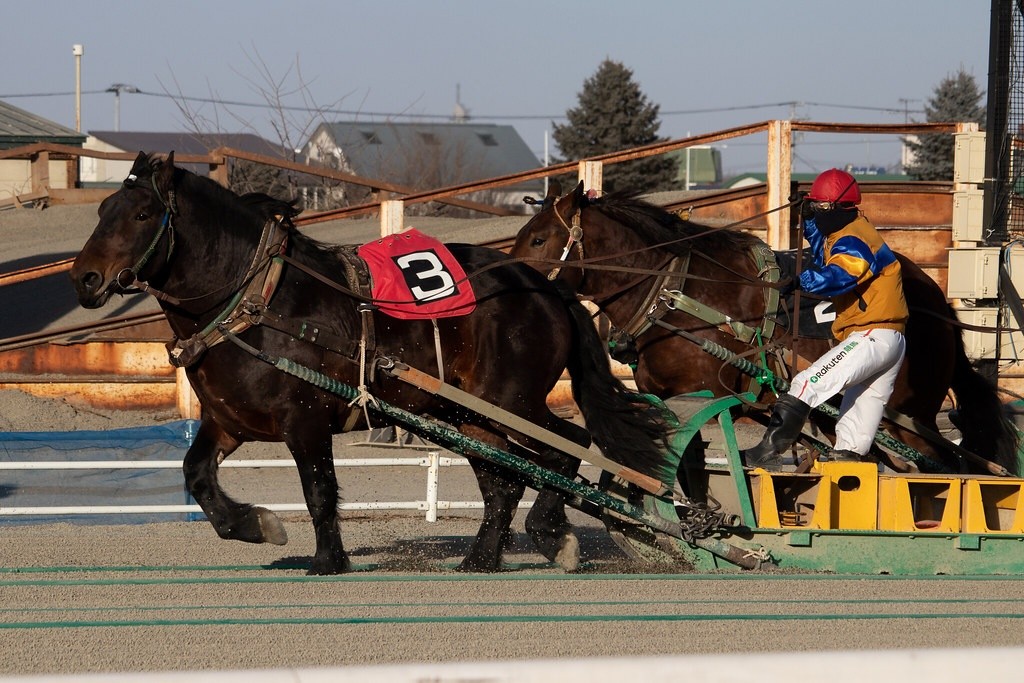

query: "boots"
[739,393,811,473]
[829,448,861,462]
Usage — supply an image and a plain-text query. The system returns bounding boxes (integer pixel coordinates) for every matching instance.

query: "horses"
[69,149,665,575]
[509,180,1023,473]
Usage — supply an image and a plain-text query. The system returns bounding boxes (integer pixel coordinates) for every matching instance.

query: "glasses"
[810,200,856,213]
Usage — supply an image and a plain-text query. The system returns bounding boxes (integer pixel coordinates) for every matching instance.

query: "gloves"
[789,191,814,220]
[783,275,801,297]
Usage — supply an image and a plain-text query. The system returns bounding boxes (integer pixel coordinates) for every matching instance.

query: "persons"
[739,168,910,472]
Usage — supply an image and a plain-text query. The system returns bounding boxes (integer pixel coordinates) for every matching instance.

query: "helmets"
[803,168,861,204]
